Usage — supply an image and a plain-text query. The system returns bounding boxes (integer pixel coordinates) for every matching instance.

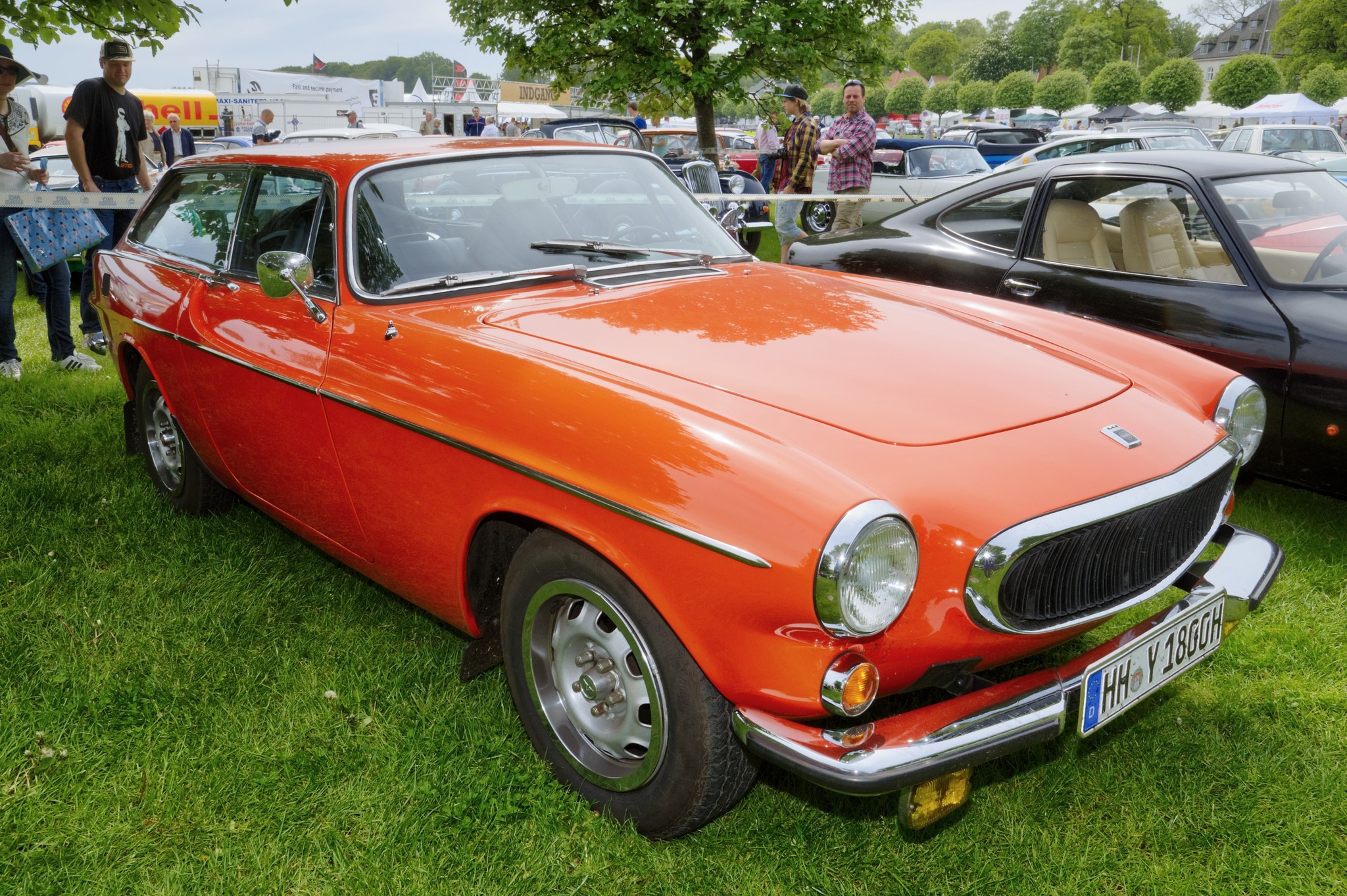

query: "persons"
[627,100,671,157]
[894,121,905,139]
[498,117,551,138]
[480,116,500,137]
[923,118,935,140]
[0,42,103,380]
[418,111,433,137]
[753,83,825,265]
[344,109,365,129]
[1328,114,1347,143]
[136,109,195,170]
[431,118,443,135]
[252,109,281,147]
[975,117,1317,131]
[815,79,876,231]
[62,34,159,349]
[465,107,487,137]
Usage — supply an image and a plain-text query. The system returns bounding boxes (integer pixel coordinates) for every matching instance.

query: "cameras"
[767,146,788,161]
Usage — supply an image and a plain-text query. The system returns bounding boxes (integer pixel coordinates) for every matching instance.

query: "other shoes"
[83,332,106,349]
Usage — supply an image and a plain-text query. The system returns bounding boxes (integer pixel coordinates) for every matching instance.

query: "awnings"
[496,101,567,119]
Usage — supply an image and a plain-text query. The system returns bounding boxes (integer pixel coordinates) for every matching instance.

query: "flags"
[313,54,328,74]
[453,60,467,102]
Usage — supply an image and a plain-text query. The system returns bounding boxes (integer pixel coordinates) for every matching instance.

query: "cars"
[92,136,1288,843]
[784,149,1347,499]
[800,121,1347,237]
[15,118,829,295]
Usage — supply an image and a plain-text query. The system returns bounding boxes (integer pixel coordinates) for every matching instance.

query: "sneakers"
[0,358,24,382]
[52,349,101,372]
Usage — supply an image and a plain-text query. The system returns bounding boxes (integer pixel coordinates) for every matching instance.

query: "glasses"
[845,80,862,86]
[170,121,177,123]
[0,64,20,75]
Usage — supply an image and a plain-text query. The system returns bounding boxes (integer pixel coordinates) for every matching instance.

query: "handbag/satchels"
[5,162,110,274]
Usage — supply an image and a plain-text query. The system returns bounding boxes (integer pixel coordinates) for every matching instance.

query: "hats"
[0,43,48,86]
[774,83,809,101]
[99,36,135,61]
[344,110,357,116]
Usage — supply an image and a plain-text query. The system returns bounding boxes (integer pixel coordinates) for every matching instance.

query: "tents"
[1012,91,1339,128]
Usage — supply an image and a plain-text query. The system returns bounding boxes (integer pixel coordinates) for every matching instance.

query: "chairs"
[1291,140,1308,150]
[1118,196,1208,281]
[279,196,574,286]
[1042,199,1116,271]
